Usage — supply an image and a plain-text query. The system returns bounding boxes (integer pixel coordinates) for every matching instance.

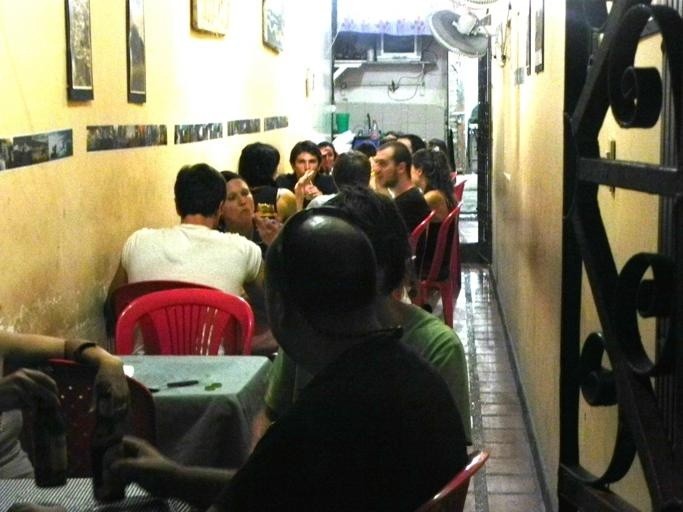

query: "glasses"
[276,207,350,288]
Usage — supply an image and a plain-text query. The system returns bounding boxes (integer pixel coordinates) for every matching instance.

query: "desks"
[101,348,274,469]
[0,470,198,511]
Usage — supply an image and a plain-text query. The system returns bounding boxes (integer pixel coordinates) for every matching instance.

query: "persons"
[101,134,473,511]
[1,329,132,480]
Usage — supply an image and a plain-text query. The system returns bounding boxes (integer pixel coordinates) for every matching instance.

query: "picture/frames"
[63,0,94,101]
[126,0,146,103]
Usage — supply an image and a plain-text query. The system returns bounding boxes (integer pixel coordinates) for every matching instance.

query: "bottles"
[31,369,68,488]
[85,381,126,502]
[369,120,378,140]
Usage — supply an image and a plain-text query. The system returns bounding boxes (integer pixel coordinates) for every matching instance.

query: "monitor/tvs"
[381,33,417,56]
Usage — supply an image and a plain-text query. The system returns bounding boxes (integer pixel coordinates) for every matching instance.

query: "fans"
[432,11,504,58]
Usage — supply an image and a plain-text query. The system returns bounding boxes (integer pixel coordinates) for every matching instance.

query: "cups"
[336,113,350,132]
[366,48,374,61]
[258,203,276,223]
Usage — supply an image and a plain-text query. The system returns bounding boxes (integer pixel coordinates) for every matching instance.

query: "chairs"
[448,170,457,183]
[453,178,467,201]
[103,281,238,353]
[420,444,488,512]
[408,208,435,309]
[421,200,461,334]
[114,290,255,355]
[38,359,155,476]
